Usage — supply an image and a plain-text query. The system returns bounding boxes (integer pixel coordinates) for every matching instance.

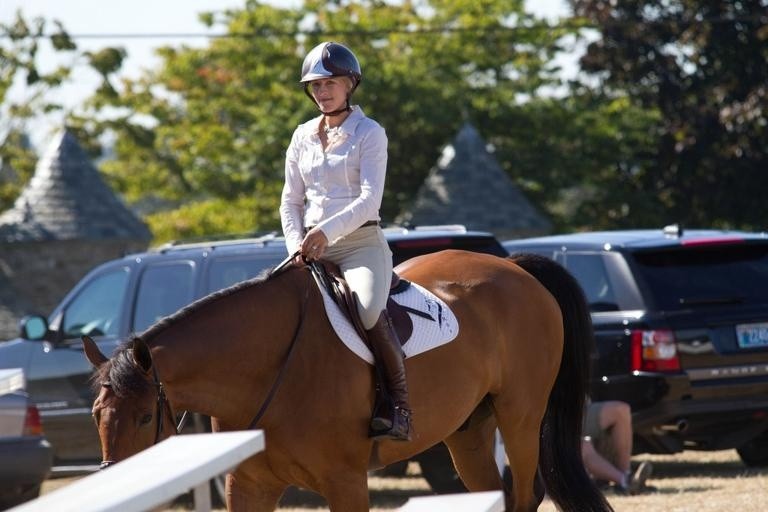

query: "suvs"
[499,222,766,466]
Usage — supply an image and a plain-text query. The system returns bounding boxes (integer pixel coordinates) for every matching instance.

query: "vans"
[0,222,511,475]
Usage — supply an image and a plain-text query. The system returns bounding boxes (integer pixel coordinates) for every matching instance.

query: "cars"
[0,368,54,512]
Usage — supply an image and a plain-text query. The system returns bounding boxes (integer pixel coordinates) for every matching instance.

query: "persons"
[581,394,653,495]
[279,41,414,439]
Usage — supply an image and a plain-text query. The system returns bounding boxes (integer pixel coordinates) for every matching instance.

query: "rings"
[312,245,318,249]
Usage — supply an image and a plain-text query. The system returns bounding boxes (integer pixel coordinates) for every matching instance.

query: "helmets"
[301,42,361,82]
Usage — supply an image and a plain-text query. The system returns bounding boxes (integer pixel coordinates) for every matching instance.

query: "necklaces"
[319,122,333,140]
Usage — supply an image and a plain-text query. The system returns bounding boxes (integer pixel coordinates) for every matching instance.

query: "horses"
[82,241,616,511]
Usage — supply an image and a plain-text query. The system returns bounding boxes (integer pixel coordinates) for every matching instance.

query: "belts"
[305,220,377,234]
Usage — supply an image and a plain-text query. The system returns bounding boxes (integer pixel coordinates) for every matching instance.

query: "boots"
[365,309,411,433]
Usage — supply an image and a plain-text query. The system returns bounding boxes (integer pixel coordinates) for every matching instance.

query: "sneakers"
[627,462,652,491]
